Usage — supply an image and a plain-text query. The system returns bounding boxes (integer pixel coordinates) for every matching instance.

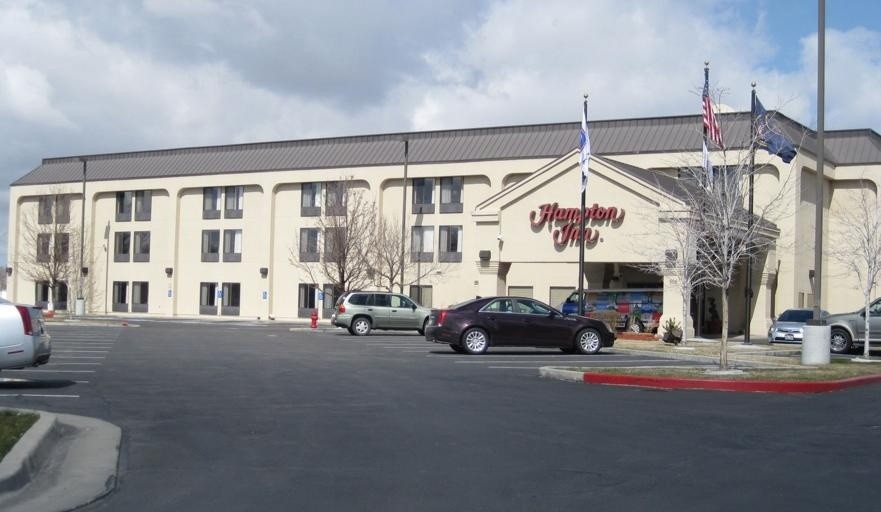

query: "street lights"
[401,138,409,293]
[76,156,89,318]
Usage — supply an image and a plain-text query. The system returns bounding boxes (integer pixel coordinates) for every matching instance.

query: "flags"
[700,67,726,151]
[578,111,591,193]
[750,93,799,163]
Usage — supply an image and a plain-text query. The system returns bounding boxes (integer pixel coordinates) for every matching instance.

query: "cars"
[425,296,618,354]
[821,297,881,354]
[0,296,52,371]
[768,308,831,345]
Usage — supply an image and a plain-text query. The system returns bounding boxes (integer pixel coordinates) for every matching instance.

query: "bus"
[563,288,697,334]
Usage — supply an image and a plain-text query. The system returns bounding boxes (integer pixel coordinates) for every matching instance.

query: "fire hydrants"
[311,311,318,328]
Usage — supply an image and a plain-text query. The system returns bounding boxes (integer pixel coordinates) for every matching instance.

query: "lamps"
[665,249,678,262]
[260,267,268,275]
[82,267,89,273]
[478,249,491,260]
[165,267,173,273]
[6,267,12,273]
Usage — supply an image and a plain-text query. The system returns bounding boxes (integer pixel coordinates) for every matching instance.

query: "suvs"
[331,290,432,336]
